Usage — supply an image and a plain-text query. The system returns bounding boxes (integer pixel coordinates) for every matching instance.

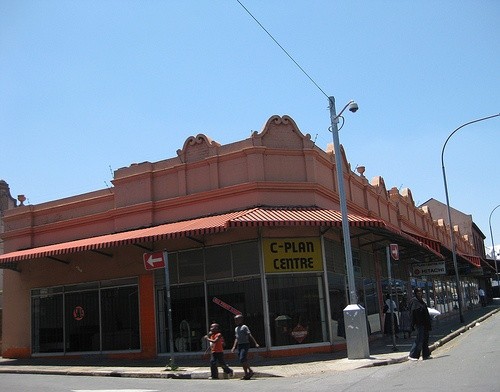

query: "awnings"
[0,208,498,273]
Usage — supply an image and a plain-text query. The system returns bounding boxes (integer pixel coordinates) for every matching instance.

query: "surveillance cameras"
[349,102,358,112]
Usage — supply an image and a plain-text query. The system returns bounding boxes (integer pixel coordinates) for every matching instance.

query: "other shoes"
[230,369,234,377]
[423,356,432,360]
[408,356,419,361]
[209,376,218,380]
[240,371,254,380]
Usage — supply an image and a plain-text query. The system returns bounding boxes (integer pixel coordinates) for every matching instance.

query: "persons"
[399,305,413,340]
[409,287,432,360]
[231,314,260,380]
[479,287,486,308]
[204,324,233,380]
[383,294,399,343]
